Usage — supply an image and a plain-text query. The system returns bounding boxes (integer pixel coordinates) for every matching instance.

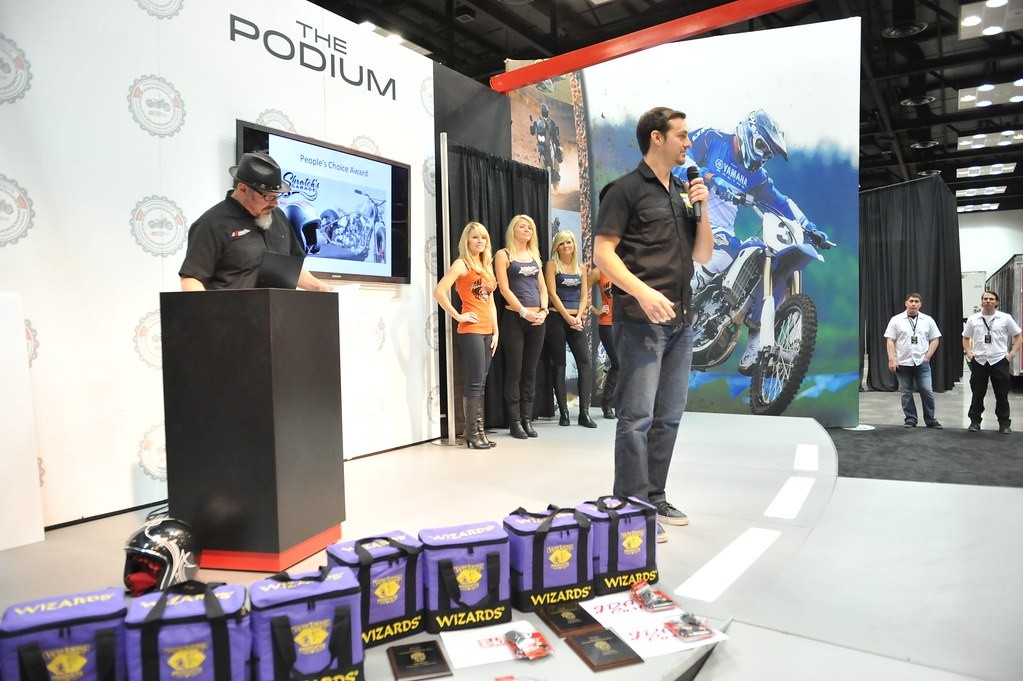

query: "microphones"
[687,166,702,223]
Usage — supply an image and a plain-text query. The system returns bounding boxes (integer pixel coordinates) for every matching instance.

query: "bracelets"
[540,308,550,315]
[519,306,527,318]
[589,304,593,311]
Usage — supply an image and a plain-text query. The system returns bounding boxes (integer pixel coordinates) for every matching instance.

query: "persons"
[593,109,715,544]
[545,230,597,428]
[179,153,332,292]
[962,291,1023,433]
[495,214,548,439]
[531,103,563,169]
[435,221,498,449]
[884,293,944,429]
[585,261,621,419]
[669,106,828,377]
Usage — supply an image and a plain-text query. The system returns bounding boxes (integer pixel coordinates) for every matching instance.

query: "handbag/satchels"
[247,567,364,681]
[418,522,512,633]
[325,531,426,648]
[575,494,658,596]
[504,504,595,612]
[0,588,127,681]
[124,579,252,681]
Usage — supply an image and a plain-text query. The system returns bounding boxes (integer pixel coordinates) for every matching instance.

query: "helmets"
[737,107,790,169]
[123,518,200,593]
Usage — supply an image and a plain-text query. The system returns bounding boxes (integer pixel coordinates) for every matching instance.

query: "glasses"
[750,126,774,162]
[247,184,283,202]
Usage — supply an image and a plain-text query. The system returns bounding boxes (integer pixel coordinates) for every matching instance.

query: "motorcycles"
[690,189,836,415]
[529,115,560,190]
[321,189,386,264]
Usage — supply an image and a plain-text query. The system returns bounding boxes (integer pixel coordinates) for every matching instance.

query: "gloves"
[807,222,828,245]
[713,176,734,201]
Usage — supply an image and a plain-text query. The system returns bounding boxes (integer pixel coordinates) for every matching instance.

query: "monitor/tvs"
[235,119,412,285]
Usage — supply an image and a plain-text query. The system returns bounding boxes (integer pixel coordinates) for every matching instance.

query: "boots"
[601,368,618,419]
[552,364,570,425]
[463,395,497,448]
[508,403,528,438]
[577,363,597,427]
[520,401,538,437]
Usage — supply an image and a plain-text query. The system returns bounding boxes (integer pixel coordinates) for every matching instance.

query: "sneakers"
[969,422,981,430]
[656,523,667,543]
[927,422,943,428]
[903,421,916,428]
[655,501,689,526]
[999,425,1011,433]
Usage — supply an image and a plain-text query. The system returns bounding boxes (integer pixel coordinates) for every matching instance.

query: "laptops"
[255,251,303,289]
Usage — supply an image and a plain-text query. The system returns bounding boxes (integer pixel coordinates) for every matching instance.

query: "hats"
[229,152,291,192]
